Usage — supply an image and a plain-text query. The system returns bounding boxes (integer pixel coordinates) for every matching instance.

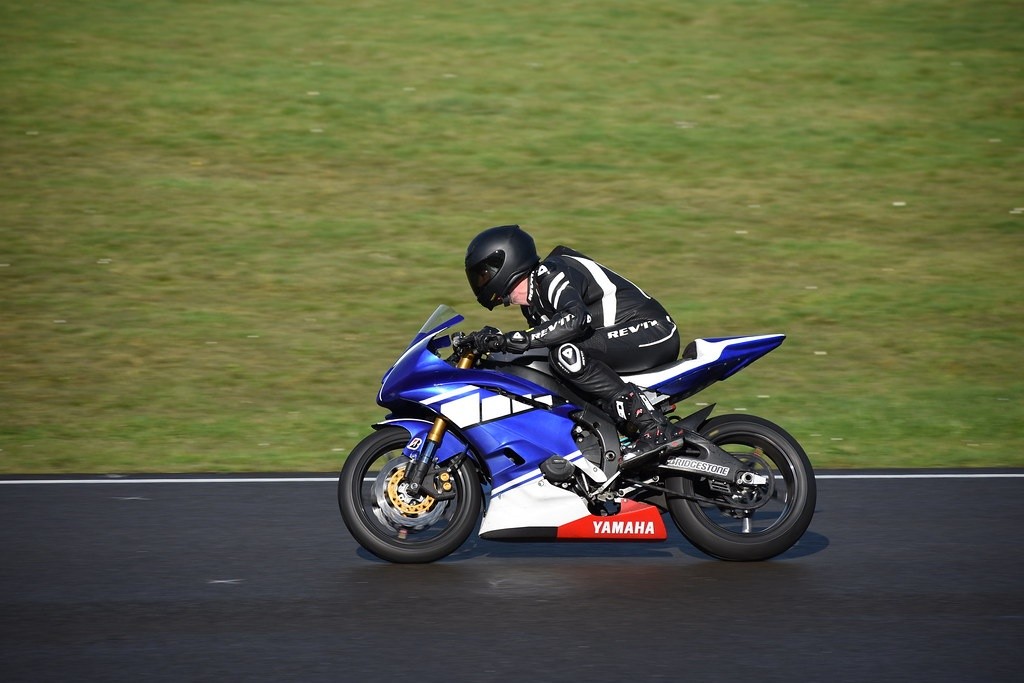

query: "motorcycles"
[337,305,817,566]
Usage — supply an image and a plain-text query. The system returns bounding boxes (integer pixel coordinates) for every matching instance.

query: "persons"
[464,225,691,468]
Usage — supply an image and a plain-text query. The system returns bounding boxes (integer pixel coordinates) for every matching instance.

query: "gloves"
[459,324,505,354]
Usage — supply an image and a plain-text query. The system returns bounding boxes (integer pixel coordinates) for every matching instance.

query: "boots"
[605,382,684,466]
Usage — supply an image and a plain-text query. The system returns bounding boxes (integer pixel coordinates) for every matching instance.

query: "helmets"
[464,224,540,309]
